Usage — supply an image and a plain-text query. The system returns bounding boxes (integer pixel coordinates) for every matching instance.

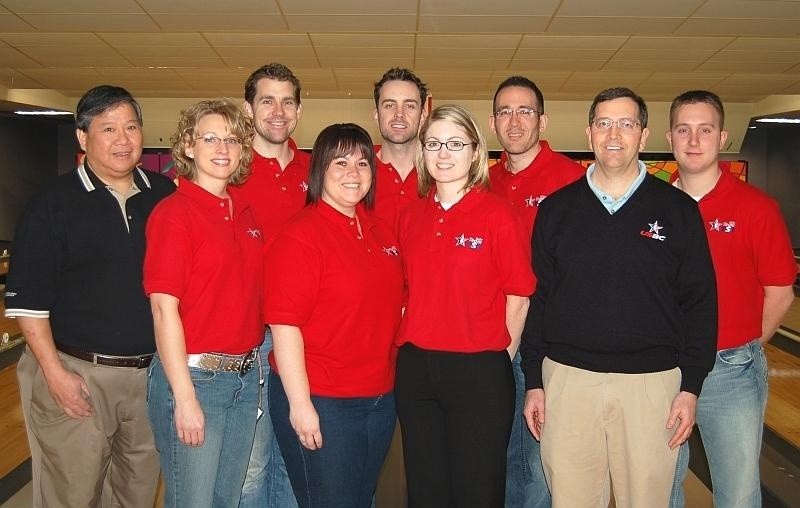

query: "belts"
[50,339,151,369]
[186,344,261,377]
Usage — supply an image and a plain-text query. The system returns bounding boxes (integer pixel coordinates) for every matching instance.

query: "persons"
[519,86,720,508]
[141,98,270,508]
[393,104,539,508]
[3,85,176,508]
[262,121,406,508]
[369,64,432,507]
[223,62,317,508]
[478,72,589,508]
[662,89,796,508]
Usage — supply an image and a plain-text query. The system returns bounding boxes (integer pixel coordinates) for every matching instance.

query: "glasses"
[194,136,242,147]
[422,140,472,150]
[594,118,641,131]
[495,109,539,119]
